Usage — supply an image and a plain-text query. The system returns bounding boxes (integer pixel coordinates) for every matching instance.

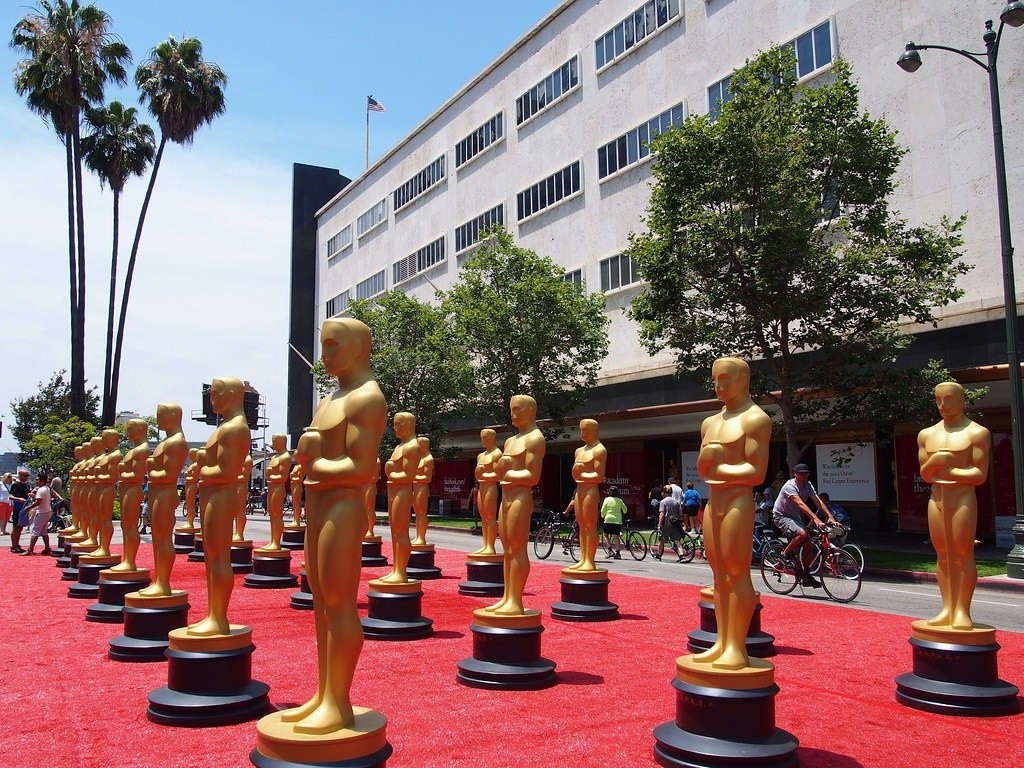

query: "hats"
[776,471,785,478]
[609,486,620,492]
[794,463,811,474]
[763,487,774,496]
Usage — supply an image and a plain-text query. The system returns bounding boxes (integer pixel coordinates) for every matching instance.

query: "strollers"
[51,499,72,533]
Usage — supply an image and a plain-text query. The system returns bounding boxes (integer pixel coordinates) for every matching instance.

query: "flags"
[367,93,385,112]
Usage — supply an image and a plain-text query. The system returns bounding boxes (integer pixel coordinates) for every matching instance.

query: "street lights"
[896,0,1024,578]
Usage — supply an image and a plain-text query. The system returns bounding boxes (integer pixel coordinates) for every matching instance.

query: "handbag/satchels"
[670,517,682,530]
[17,497,30,526]
[698,509,704,528]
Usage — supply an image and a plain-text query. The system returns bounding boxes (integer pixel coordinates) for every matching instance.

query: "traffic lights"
[256,463,261,469]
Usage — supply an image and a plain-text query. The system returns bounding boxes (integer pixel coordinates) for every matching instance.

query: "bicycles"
[120,506,176,529]
[601,519,647,561]
[752,525,785,568]
[647,515,695,564]
[183,497,200,517]
[533,509,581,563]
[760,523,864,604]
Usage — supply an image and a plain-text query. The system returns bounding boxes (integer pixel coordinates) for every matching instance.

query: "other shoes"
[139,529,146,534]
[19,550,32,556]
[474,527,478,529]
[10,545,25,553]
[688,531,696,534]
[605,552,614,559]
[614,554,621,559]
[39,548,51,555]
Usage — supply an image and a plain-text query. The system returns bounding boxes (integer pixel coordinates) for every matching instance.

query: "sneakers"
[651,553,661,561]
[803,576,821,588]
[676,555,686,563]
[773,558,796,575]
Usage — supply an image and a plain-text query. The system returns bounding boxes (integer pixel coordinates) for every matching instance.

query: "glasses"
[21,474,29,476]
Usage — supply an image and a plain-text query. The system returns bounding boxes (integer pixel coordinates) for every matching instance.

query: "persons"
[233,453,252,546]
[0,468,70,556]
[380,412,435,583]
[260,433,306,550]
[572,418,607,572]
[692,356,772,670]
[563,480,628,561]
[281,316,387,735]
[57,376,199,599]
[754,464,850,588]
[475,394,546,615]
[260,486,267,516]
[647,456,703,564]
[247,485,260,504]
[185,382,252,635]
[466,479,481,530]
[917,382,991,631]
[138,476,152,535]
